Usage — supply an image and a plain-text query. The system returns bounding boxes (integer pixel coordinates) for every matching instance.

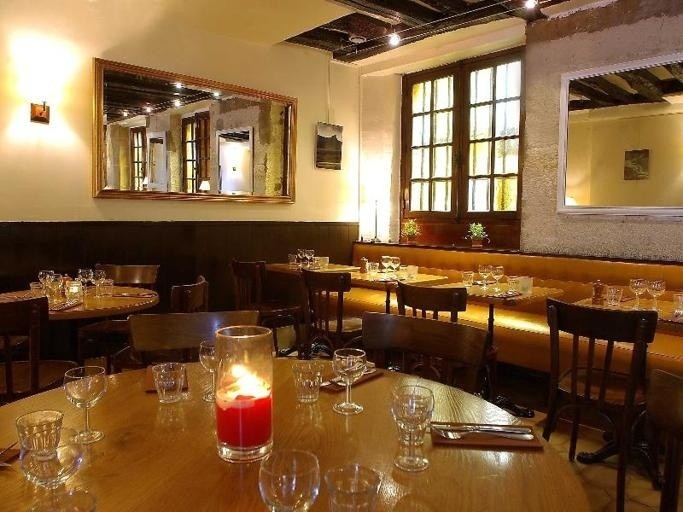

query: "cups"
[461,271,473,287]
[210,324,274,463]
[149,361,185,404]
[388,385,434,470]
[259,446,321,511]
[289,361,322,402]
[360,257,367,272]
[591,280,604,300]
[15,409,63,462]
[325,463,380,512]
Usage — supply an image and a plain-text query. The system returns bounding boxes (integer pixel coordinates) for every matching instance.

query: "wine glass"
[29,269,112,303]
[332,346,368,415]
[477,264,533,295]
[367,256,418,279]
[607,279,682,314]
[18,426,96,512]
[287,248,330,269]
[199,340,219,402]
[61,365,108,444]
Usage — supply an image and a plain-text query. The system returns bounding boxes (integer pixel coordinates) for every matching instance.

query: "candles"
[216,364,271,448]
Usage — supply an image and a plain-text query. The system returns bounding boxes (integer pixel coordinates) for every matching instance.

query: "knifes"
[432,422,532,433]
[319,362,366,387]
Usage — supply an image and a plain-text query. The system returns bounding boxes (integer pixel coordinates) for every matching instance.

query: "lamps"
[197,180,210,193]
[142,176,151,190]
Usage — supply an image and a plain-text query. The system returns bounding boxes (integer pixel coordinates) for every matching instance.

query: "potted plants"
[464,222,487,249]
[400,220,422,246]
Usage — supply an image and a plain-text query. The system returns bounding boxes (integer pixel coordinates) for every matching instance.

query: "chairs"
[1,265,259,404]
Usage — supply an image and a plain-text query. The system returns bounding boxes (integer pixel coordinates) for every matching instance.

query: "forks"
[431,425,534,440]
[329,368,378,387]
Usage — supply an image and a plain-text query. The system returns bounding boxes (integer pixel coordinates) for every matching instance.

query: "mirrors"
[92,57,297,203]
[555,51,683,223]
